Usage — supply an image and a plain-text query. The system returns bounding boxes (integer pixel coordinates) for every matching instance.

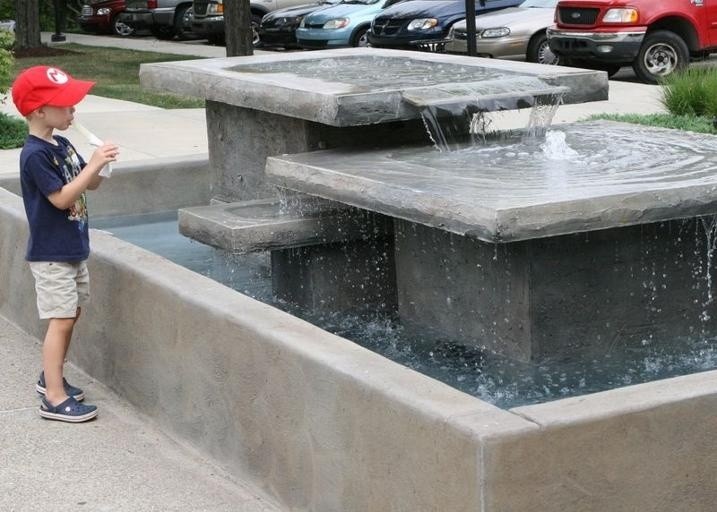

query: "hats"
[10,64,97,115]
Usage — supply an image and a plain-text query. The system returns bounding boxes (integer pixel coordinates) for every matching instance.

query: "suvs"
[544,1,716,86]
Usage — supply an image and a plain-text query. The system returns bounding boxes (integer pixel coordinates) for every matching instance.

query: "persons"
[12,65,120,424]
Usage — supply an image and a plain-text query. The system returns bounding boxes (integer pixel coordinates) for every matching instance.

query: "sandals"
[39,396,99,422]
[36,375,85,403]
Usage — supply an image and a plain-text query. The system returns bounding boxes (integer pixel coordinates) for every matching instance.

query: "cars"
[364,1,525,54]
[258,1,355,52]
[188,1,319,48]
[295,1,402,51]
[77,1,136,38]
[444,1,563,66]
[119,1,208,41]
